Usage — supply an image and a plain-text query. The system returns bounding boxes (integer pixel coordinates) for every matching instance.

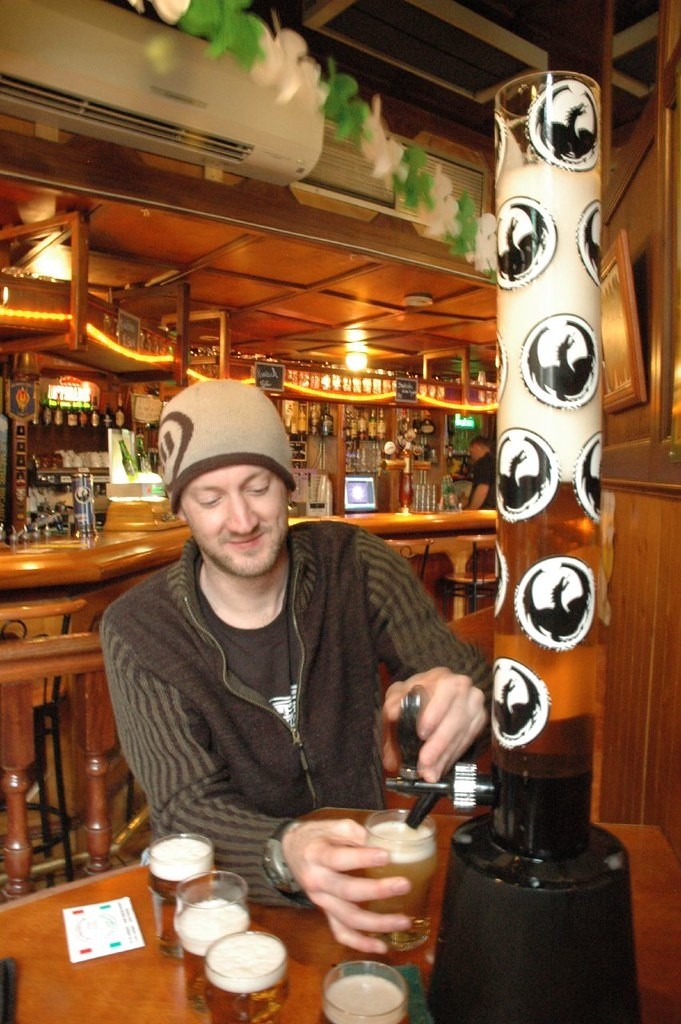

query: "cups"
[204,930,289,1024]
[320,960,412,1024]
[147,832,215,960]
[173,869,252,1008]
[365,808,439,955]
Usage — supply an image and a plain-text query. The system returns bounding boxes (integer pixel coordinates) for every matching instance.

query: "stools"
[0,598,88,888]
[386,538,435,583]
[442,534,494,616]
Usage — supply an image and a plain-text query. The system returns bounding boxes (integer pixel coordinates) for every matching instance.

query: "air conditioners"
[0,0,325,186]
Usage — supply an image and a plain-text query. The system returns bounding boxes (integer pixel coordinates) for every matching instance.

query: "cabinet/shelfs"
[345,438,386,477]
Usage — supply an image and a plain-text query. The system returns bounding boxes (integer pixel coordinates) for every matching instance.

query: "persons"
[465,435,493,511]
[100,379,493,954]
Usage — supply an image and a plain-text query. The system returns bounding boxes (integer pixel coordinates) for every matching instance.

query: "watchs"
[261,819,302,897]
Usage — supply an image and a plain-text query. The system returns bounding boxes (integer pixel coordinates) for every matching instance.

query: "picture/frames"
[599,229,647,415]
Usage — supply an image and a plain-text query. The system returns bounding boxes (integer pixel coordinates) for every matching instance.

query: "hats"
[158,380,296,515]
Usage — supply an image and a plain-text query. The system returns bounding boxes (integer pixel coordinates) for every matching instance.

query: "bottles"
[40,391,125,429]
[280,400,460,512]
[119,435,151,477]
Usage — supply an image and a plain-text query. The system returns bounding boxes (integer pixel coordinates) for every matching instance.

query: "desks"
[0,806,681,1024]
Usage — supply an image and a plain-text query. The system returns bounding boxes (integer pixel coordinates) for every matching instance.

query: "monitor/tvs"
[344,475,377,513]
